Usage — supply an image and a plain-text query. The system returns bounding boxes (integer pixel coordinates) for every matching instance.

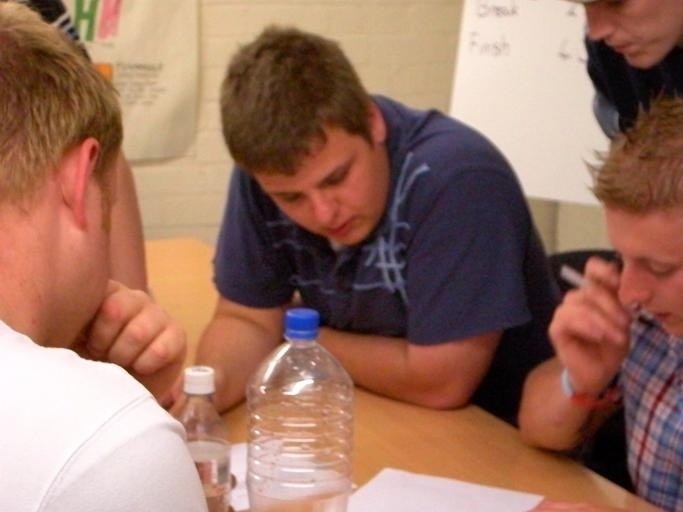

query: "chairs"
[547,246,622,300]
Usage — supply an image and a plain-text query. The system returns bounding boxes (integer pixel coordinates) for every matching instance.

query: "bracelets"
[559,367,628,411]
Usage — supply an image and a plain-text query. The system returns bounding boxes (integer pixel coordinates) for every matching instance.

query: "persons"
[17,0,145,294]
[578,0,681,143]
[161,21,573,432]
[515,82,681,512]
[2,1,237,512]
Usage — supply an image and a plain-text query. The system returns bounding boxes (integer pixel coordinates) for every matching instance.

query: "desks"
[143,238,667,511]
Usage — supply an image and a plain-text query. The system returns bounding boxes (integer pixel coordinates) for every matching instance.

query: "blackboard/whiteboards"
[449,0,604,206]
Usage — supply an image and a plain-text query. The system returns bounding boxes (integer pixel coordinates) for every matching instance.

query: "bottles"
[246,308,356,512]
[173,367,230,512]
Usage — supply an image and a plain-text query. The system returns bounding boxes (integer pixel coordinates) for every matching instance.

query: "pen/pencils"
[560,265,654,328]
